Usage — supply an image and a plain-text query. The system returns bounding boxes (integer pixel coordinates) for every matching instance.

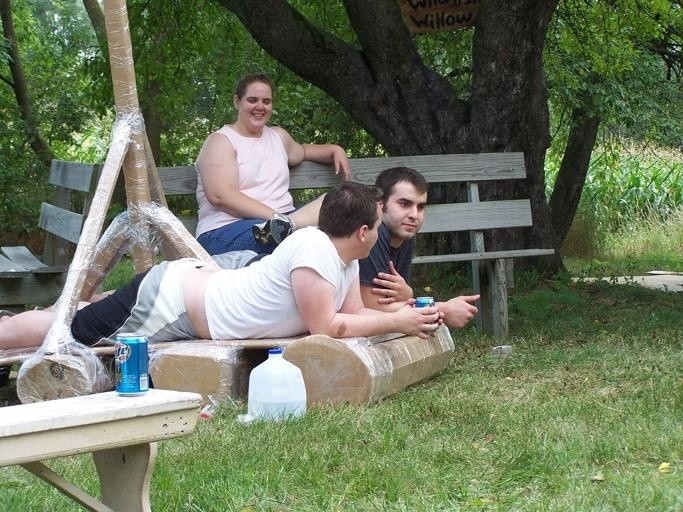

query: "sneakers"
[253,213,296,246]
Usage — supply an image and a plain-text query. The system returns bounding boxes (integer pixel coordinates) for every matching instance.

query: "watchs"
[271,212,280,220]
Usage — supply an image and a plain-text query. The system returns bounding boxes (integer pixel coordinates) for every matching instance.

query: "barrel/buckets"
[248,347,307,420]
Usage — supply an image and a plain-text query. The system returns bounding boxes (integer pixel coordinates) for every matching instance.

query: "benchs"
[0,389,201,511]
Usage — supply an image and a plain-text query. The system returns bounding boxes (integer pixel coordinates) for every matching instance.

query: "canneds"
[416,296,436,337]
[115,331,149,396]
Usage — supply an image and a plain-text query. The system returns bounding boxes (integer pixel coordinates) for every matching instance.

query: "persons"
[0,181,445,350]
[194,73,352,258]
[213,167,481,330]
